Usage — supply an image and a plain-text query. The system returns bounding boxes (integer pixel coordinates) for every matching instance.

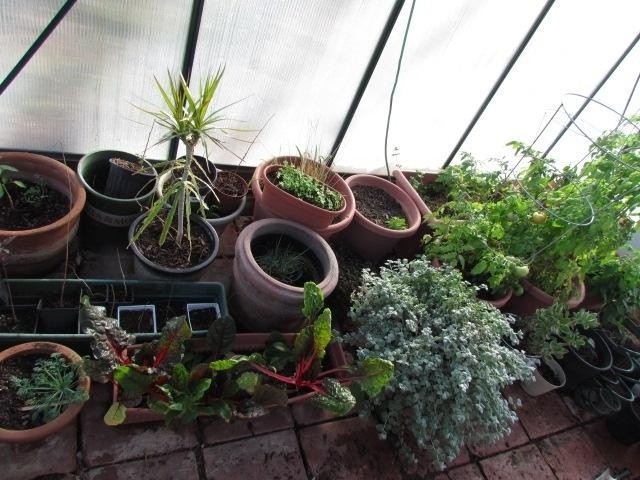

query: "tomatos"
[515,285,525,297]
[533,211,548,224]
[516,265,529,278]
